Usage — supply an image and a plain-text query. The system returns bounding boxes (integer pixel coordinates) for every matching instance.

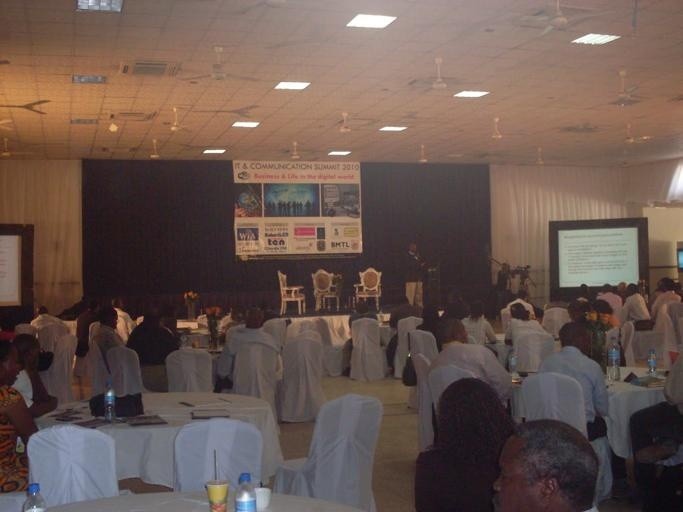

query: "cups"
[205,482,228,512]
[253,488,270,512]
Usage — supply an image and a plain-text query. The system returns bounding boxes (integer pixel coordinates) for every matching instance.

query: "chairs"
[310,269,340,312]
[352,267,383,311]
[277,270,307,316]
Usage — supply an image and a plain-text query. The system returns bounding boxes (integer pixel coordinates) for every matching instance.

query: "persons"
[2,284,180,366]
[197,284,283,393]
[0,338,39,493]
[386,277,682,511]
[341,300,384,378]
[235,190,360,218]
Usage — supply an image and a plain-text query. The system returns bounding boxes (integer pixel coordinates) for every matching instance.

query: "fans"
[1,135,36,158]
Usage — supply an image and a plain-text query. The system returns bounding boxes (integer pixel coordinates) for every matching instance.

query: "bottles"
[647,349,657,376]
[103,380,116,424]
[22,483,47,512]
[508,350,518,379]
[608,337,621,381]
[234,474,257,512]
